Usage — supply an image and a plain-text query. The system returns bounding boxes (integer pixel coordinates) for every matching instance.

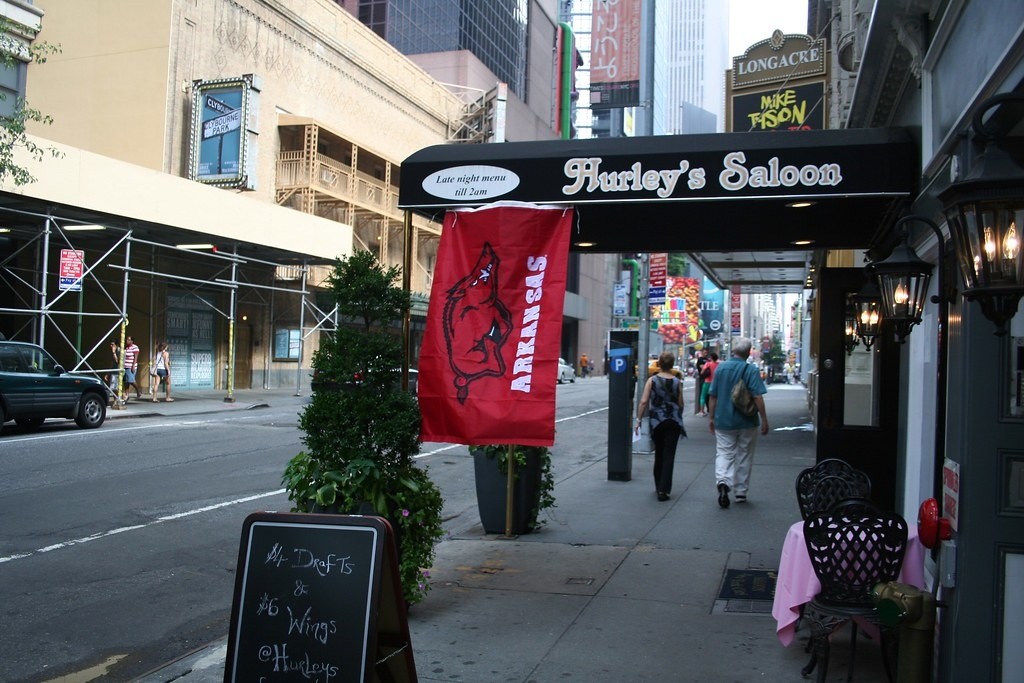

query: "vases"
[306,497,409,609]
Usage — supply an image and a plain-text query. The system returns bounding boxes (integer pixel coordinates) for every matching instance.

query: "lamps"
[936,92,1024,338]
[865,215,957,344]
[845,275,884,356]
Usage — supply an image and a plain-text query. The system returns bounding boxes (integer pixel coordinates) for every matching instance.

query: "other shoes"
[657,488,669,500]
[137,390,143,399]
[152,398,160,403]
[123,396,129,402]
[696,410,708,417]
[718,484,730,507]
[736,496,746,502]
[166,397,174,402]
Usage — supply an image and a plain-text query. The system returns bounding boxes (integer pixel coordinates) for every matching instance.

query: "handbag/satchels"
[702,369,710,378]
[150,364,158,377]
[731,362,759,417]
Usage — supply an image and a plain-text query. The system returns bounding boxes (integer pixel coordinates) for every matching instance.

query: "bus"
[759,360,772,378]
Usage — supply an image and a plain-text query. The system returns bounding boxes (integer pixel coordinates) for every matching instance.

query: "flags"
[419,202,574,447]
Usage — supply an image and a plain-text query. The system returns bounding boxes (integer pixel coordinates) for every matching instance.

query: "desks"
[772,517,926,683]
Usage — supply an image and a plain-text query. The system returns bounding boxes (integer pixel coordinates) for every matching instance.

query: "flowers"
[282,243,450,609]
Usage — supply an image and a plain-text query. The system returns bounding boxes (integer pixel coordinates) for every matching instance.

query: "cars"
[634,360,682,381]
[557,358,576,384]
[0,340,109,429]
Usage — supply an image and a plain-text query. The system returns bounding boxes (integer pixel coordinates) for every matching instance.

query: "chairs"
[795,458,908,683]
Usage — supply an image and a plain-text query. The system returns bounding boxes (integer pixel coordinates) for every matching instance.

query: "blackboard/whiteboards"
[222,511,419,683]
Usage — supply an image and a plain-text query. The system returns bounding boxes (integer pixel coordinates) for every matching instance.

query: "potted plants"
[468,445,560,534]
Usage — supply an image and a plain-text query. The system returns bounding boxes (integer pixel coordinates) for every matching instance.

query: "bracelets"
[636,418,641,424]
[112,352,116,354]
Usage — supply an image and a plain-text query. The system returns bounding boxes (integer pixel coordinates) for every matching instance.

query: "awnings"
[396,126,921,540]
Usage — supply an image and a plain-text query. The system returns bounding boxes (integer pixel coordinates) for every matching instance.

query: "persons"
[153,341,175,402]
[123,336,142,404]
[105,337,129,406]
[708,337,770,508]
[634,351,689,501]
[580,352,589,378]
[694,348,719,417]
